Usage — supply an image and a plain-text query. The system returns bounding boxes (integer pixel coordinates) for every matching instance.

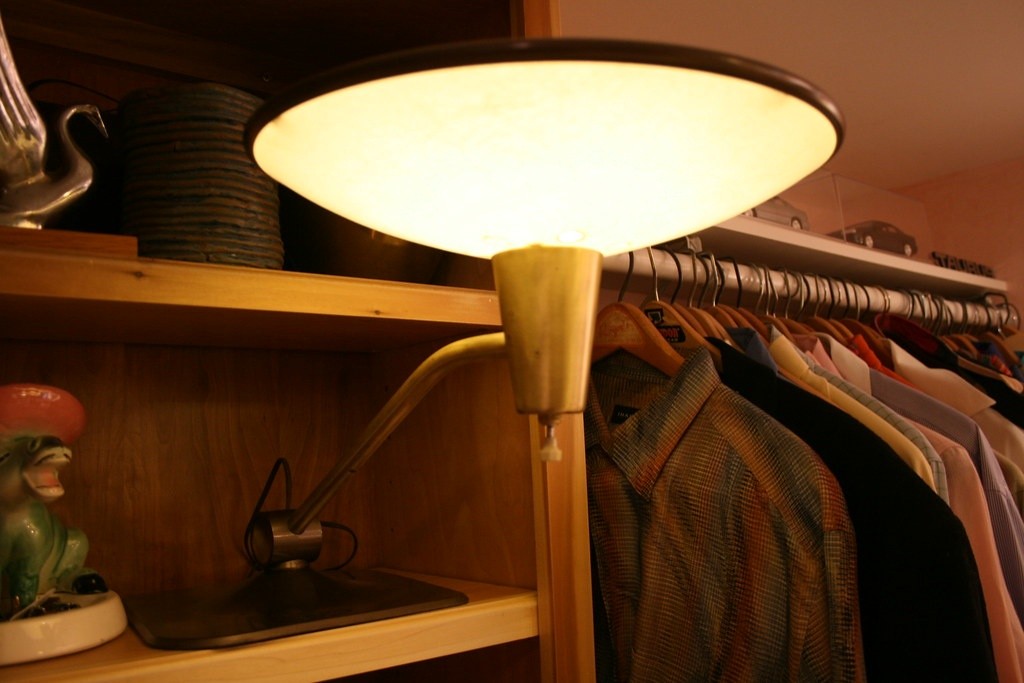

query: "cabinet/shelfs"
[0,0,597,683]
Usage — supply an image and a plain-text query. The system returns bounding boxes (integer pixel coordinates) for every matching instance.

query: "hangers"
[590,244,1020,380]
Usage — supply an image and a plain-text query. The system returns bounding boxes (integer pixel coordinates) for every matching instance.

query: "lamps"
[122,37,846,648]
